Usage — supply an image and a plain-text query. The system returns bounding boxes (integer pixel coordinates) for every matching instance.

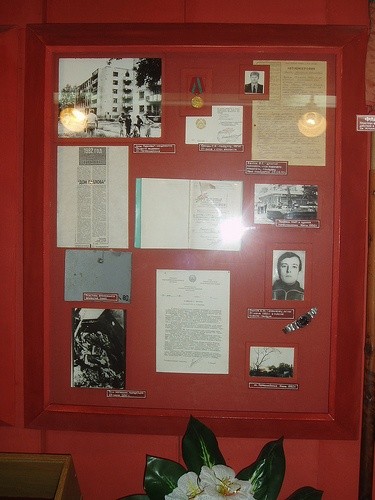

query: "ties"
[253,85,256,93]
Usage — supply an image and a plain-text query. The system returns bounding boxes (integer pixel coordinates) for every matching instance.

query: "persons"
[245,70,264,95]
[82,108,153,137]
[272,250,306,301]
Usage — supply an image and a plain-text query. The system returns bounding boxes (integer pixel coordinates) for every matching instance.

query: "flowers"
[117,413,324,500]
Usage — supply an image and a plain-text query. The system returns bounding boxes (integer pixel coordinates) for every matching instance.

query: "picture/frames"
[23,23,368,442]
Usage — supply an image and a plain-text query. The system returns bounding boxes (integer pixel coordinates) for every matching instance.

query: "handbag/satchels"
[84,128,86,132]
